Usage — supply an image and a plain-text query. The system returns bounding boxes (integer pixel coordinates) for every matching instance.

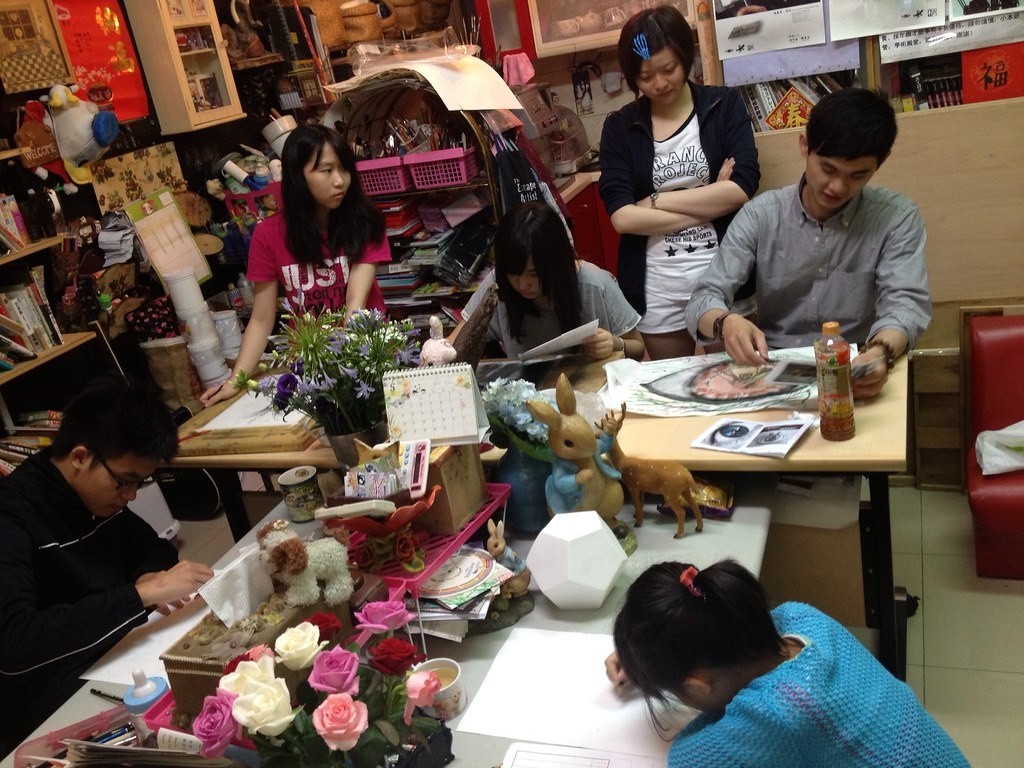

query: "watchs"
[713,311,735,343]
[650,193,658,209]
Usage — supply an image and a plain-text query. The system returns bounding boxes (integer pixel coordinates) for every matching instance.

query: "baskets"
[403,143,482,189]
[356,156,415,195]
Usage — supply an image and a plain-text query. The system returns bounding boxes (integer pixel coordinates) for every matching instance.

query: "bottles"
[206,208,265,264]
[814,321,856,442]
[278,466,324,524]
[99,295,112,314]
[243,158,256,173]
[238,272,254,305]
[224,160,257,193]
[222,168,252,203]
[35,166,60,188]
[124,667,172,748]
[254,163,271,189]
[186,339,228,381]
[176,301,216,345]
[212,311,242,350]
[204,368,232,391]
[162,267,204,310]
[227,283,245,307]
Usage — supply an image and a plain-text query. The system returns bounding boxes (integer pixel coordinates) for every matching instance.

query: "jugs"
[576,10,602,33]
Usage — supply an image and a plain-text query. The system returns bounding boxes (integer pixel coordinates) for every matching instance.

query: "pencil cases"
[14,703,141,768]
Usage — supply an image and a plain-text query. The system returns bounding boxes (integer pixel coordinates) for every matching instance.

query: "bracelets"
[619,338,625,351]
[860,339,894,369]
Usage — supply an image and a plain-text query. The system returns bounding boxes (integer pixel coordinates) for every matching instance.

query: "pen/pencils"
[90,688,124,701]
[618,682,624,699]
[387,115,422,151]
[35,721,137,768]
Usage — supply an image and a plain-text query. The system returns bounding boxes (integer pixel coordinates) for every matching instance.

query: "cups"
[600,7,626,30]
[556,18,579,39]
[409,657,468,722]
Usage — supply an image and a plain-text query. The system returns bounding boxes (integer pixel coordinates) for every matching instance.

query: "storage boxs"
[403,147,480,190]
[420,445,489,536]
[89,141,187,217]
[355,156,412,195]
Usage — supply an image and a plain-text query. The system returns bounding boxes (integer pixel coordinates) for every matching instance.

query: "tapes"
[261,115,297,144]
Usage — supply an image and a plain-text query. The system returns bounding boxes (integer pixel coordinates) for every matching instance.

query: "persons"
[444,201,645,363]
[685,85,932,400]
[200,123,392,408]
[605,558,972,768]
[0,382,214,763]
[597,4,762,361]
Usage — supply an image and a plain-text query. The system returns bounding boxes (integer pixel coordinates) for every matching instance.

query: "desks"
[561,172,620,278]
[152,282,494,543]
[482,354,909,682]
[158,436,339,543]
[0,499,772,768]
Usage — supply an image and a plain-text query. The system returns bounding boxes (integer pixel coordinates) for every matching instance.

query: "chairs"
[960,315,1024,579]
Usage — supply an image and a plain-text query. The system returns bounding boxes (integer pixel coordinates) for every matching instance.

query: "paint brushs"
[427,112,453,150]
[459,15,481,53]
[489,46,502,75]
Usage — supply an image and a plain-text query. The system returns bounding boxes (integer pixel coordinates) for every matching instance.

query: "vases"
[325,421,388,468]
[493,447,553,536]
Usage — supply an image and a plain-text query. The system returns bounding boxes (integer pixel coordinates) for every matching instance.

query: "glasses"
[98,457,155,492]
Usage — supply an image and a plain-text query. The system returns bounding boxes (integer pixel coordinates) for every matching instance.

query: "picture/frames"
[0,0,76,95]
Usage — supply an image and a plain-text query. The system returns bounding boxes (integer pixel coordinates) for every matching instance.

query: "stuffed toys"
[256,519,354,608]
[419,316,457,366]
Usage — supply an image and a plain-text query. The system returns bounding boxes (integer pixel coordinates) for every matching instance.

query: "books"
[910,72,962,111]
[0,194,31,253]
[0,265,64,369]
[690,418,815,458]
[0,410,62,476]
[735,74,843,133]
[373,197,495,327]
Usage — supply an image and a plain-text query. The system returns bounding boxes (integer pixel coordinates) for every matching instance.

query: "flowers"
[191,600,441,768]
[228,290,421,428]
[480,376,558,445]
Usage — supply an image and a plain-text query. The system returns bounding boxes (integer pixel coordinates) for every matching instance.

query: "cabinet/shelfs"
[474,0,1024,132]
[0,146,95,434]
[123,0,248,135]
